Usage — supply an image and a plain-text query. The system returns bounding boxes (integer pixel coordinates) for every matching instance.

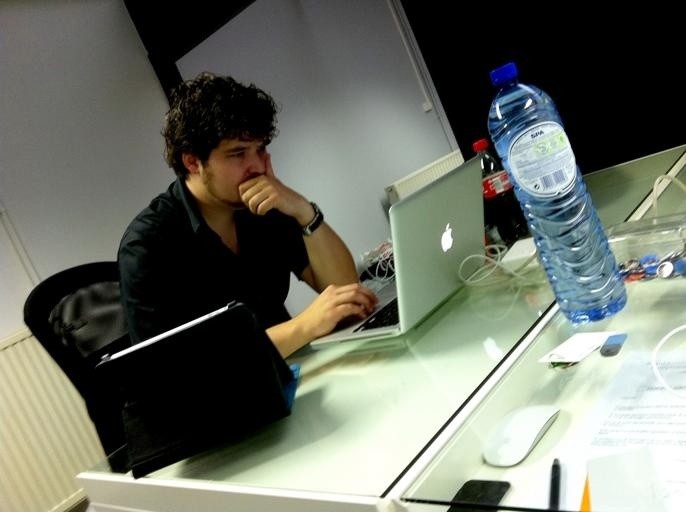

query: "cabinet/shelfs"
[472,139,531,251]
[486,63,629,326]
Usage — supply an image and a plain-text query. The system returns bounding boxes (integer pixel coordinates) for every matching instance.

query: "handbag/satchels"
[302,200,323,235]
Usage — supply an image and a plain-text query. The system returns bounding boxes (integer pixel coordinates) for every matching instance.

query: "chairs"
[23,261,132,473]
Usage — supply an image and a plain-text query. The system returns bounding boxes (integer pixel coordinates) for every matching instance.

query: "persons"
[115,71,380,375]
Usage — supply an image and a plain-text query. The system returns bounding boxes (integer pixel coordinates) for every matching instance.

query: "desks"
[76,143,686,511]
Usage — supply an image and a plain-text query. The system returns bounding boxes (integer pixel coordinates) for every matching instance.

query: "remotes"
[482,404,560,467]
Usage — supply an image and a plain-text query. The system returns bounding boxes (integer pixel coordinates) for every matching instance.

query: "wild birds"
[308,154,486,349]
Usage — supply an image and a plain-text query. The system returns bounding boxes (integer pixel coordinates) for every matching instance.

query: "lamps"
[23,261,132,473]
